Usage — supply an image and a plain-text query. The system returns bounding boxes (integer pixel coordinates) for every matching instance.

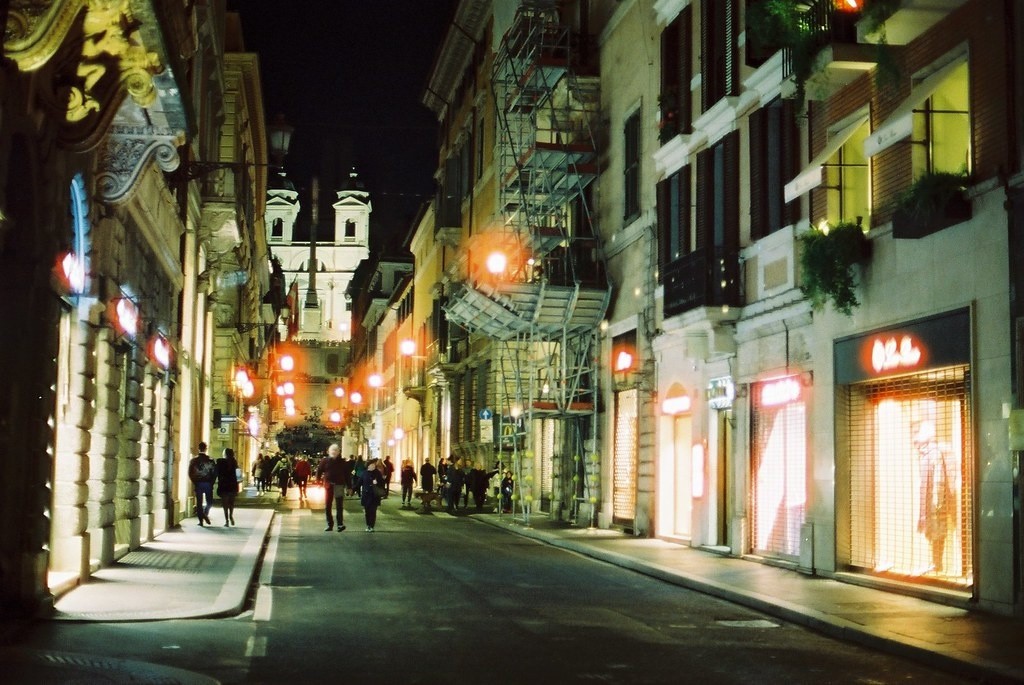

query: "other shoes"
[365,526,374,531]
[338,526,345,532]
[202,513,210,524]
[260,492,264,496]
[197,523,203,526]
[402,501,405,504]
[255,492,260,496]
[324,526,332,531]
[224,523,229,527]
[436,502,485,513]
[408,502,411,506]
[230,517,234,525]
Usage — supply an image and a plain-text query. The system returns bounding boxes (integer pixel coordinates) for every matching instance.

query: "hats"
[364,460,376,468]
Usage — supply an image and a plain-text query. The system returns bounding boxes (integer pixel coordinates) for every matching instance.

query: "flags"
[287,277,299,340]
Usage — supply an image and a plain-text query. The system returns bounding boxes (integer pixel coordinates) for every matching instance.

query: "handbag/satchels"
[334,484,345,498]
[289,478,293,488]
[372,485,386,497]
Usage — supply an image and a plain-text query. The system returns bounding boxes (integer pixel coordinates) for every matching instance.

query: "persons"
[251,451,394,499]
[316,443,351,532]
[212,448,238,526]
[351,461,384,532]
[401,456,513,513]
[189,442,215,525]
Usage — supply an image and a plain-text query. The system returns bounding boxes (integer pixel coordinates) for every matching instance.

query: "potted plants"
[893,170,974,240]
[795,222,871,319]
[747,0,816,127]
